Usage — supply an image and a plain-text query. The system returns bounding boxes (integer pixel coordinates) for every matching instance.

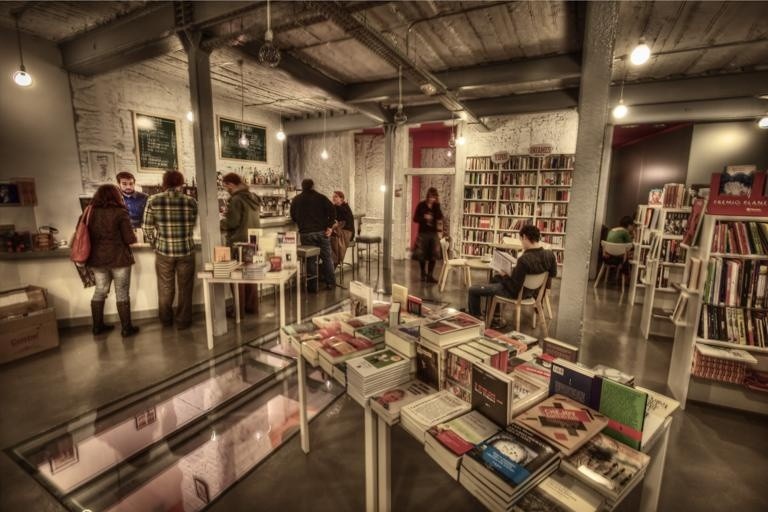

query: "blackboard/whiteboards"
[131,109,179,172]
[216,113,268,163]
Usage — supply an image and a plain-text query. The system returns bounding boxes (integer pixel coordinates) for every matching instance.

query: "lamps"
[9,10,33,87]
[394,65,631,146]
[257,2,280,69]
[235,60,249,148]
[276,98,329,161]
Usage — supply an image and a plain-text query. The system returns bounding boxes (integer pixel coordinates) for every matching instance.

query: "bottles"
[249,230,256,244]
[216,163,297,221]
[276,231,295,244]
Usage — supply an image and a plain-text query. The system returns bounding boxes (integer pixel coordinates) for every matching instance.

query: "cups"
[133,227,144,243]
[483,252,490,260]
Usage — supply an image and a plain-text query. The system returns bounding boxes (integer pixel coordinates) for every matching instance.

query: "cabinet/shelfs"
[142,186,197,198]
[459,154,576,280]
[217,185,303,218]
[627,205,768,415]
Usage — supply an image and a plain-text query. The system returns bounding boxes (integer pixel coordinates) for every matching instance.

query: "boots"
[91,300,114,335]
[116,300,139,337]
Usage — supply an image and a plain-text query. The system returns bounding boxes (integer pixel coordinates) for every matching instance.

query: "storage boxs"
[1,289,59,364]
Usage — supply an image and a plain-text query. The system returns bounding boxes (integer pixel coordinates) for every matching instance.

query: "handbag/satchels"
[70,223,92,262]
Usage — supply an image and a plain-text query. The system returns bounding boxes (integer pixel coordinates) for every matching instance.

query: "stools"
[356,236,381,284]
[336,241,355,290]
[297,244,321,301]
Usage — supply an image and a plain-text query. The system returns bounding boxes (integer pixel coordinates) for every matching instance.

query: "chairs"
[594,240,633,294]
[486,270,549,337]
[438,238,472,291]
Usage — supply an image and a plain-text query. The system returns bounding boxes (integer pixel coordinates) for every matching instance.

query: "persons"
[460,226,558,322]
[330,190,355,273]
[70,182,140,340]
[408,187,444,285]
[605,214,635,288]
[370,388,404,410]
[218,173,265,321]
[116,171,150,231]
[290,177,337,297]
[141,168,199,331]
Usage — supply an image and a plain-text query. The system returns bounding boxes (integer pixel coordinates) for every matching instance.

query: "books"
[424,411,503,481]
[197,228,298,283]
[459,423,562,511]
[283,280,486,408]
[510,469,607,512]
[370,379,439,426]
[401,389,472,444]
[633,162,768,397]
[563,433,651,509]
[461,154,575,278]
[474,326,680,459]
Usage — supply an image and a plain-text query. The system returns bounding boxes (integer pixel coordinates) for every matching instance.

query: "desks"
[203,259,301,350]
[465,258,495,314]
[280,291,682,511]
